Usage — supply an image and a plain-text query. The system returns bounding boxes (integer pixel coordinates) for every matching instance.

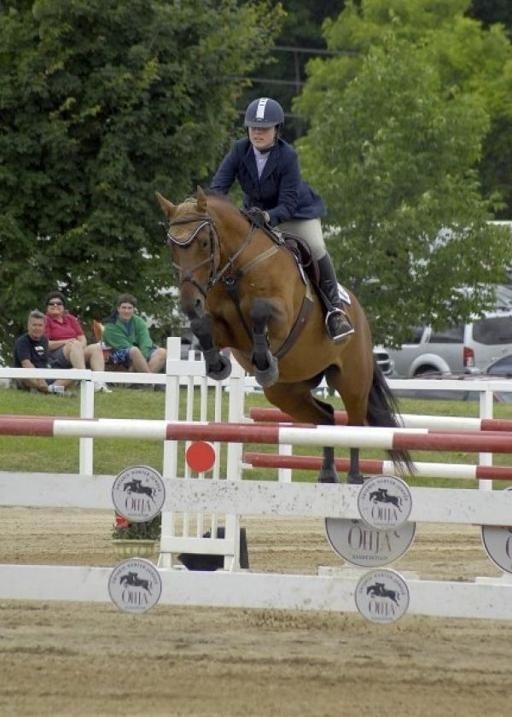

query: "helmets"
[244,96,285,129]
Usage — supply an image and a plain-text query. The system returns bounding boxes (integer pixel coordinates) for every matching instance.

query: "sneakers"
[94,382,104,393]
[47,384,65,396]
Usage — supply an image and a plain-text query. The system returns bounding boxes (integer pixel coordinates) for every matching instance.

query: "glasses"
[48,300,63,305]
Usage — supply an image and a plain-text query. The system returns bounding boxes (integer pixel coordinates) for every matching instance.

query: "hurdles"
[1,406,512,620]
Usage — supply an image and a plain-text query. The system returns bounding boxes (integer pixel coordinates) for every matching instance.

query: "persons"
[209,95,356,345]
[103,292,166,392]
[13,308,79,397]
[41,289,114,395]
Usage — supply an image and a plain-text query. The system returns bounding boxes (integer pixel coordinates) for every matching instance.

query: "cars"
[370,272,512,378]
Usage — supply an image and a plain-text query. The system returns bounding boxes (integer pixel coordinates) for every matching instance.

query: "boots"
[312,252,354,342]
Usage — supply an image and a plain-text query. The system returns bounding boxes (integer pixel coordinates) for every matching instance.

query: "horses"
[154,185,420,484]
